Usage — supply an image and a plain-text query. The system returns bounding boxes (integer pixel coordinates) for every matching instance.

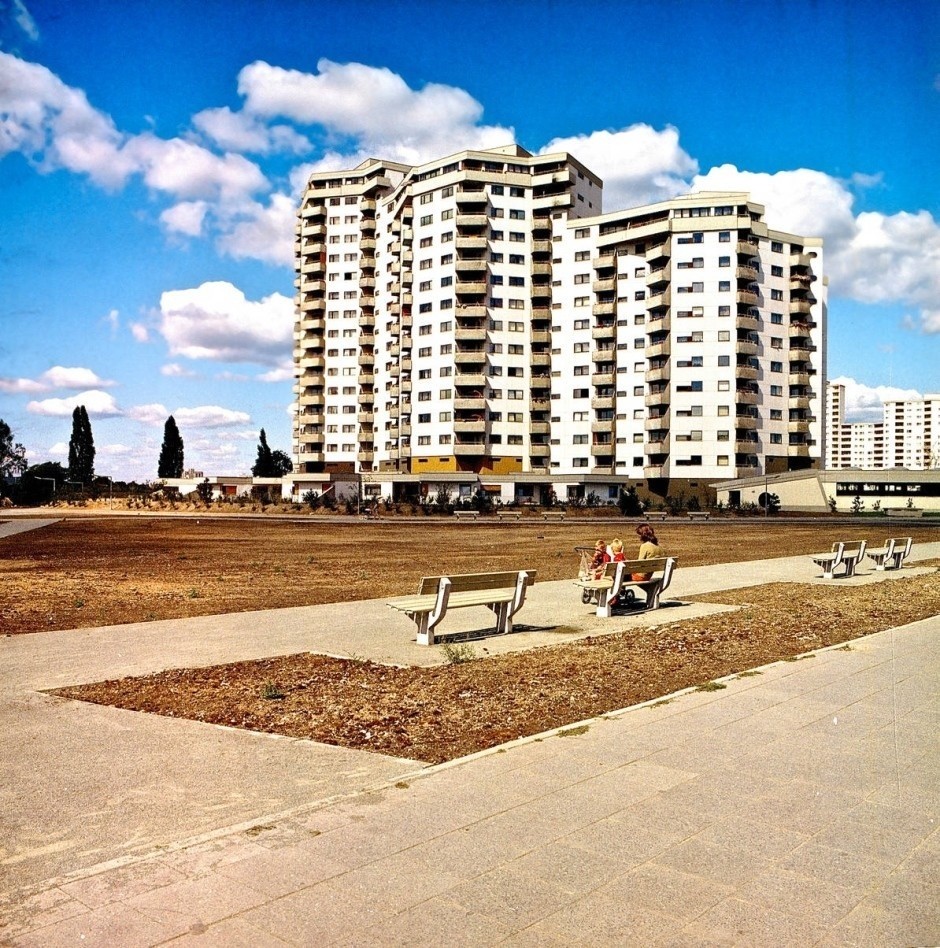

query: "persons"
[591,540,610,579]
[631,523,661,581]
[611,538,632,608]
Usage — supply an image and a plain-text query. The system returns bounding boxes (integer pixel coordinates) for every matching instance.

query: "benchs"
[865,536,913,571]
[453,510,481,522]
[385,569,537,645]
[497,511,522,521]
[572,557,679,618]
[686,512,711,521]
[643,512,667,521]
[540,511,566,522]
[808,540,867,580]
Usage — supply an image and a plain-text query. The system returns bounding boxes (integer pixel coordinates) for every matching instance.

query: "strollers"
[575,546,636,603]
